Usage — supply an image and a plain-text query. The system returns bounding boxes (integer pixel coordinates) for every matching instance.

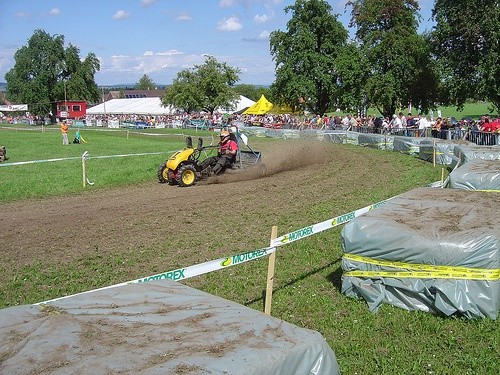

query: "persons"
[198,131,238,171]
[61,121,69,146]
[75,112,500,146]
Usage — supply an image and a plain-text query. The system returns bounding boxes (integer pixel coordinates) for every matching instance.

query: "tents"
[86,95,256,117]
[243,93,286,116]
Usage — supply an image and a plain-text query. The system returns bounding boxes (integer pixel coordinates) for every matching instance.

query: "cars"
[157,125,262,188]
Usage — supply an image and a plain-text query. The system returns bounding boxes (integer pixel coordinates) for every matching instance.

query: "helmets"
[219,131,230,143]
